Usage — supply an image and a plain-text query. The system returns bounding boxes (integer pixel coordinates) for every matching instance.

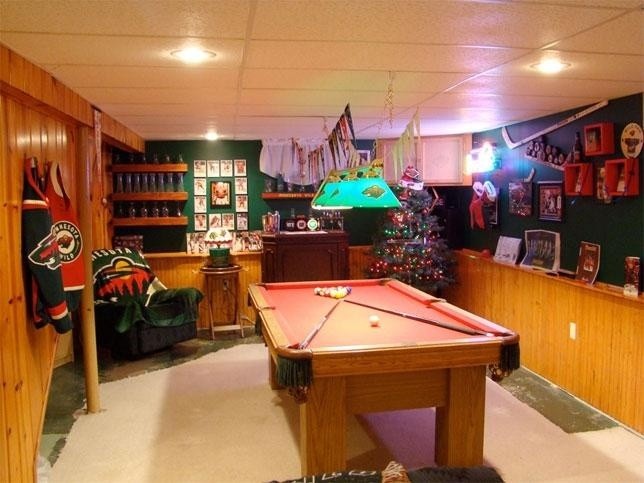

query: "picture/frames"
[357,149,370,163]
[210,181,231,209]
[537,180,565,223]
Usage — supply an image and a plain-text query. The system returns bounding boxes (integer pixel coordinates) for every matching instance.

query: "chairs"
[93,247,204,362]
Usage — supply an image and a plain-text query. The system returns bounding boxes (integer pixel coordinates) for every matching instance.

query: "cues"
[298,301,340,349]
[343,300,493,336]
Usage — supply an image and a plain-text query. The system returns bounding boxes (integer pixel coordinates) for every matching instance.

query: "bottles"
[277,172,284,192]
[111,152,185,219]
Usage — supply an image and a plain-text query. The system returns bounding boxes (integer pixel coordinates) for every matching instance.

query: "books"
[623,255,640,296]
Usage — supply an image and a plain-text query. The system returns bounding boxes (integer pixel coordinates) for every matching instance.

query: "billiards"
[315,285,351,299]
[369,315,380,327]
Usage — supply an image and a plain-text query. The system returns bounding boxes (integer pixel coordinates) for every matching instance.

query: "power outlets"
[223,280,229,290]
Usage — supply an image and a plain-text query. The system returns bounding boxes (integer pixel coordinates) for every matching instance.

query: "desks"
[199,264,245,339]
[247,277,521,477]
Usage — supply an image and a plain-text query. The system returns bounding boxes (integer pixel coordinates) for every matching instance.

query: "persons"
[193,160,247,228]
[625,131,640,153]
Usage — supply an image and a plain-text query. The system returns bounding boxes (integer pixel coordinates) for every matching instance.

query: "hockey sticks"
[524,152,573,171]
[510,168,536,183]
[501,100,609,150]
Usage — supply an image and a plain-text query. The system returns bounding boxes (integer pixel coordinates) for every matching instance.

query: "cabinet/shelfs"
[261,229,349,283]
[376,133,472,186]
[106,162,189,226]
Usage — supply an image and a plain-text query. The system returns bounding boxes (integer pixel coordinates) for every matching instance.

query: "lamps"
[311,72,404,210]
[203,227,232,267]
